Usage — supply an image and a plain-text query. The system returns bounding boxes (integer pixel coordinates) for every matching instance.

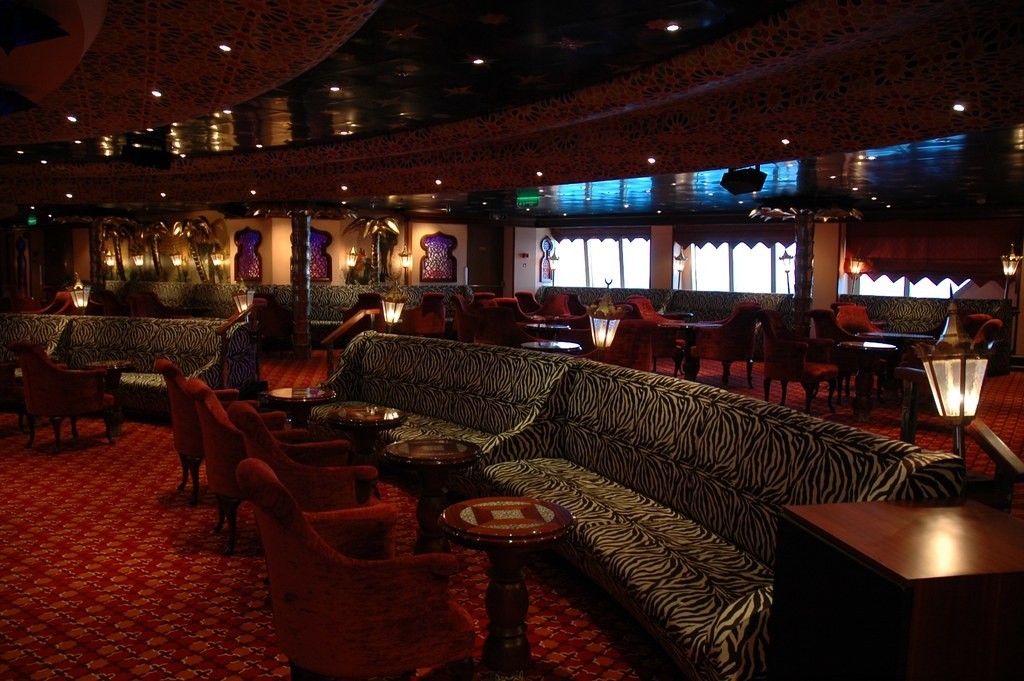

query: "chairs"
[184,378,352,556]
[234,459,476,681]
[347,291,1004,426]
[153,360,259,506]
[106,283,472,329]
[229,401,379,513]
[8,341,117,452]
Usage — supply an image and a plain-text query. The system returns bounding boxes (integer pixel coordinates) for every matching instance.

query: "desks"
[839,342,897,405]
[773,501,1024,681]
[521,341,582,355]
[321,405,405,507]
[438,497,572,671]
[525,323,571,341]
[86,359,134,436]
[855,332,936,363]
[658,323,723,381]
[382,438,481,558]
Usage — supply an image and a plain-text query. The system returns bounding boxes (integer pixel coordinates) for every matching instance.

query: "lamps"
[924,290,992,454]
[232,277,254,322]
[719,165,767,195]
[999,244,1022,300]
[378,282,410,334]
[587,281,619,363]
[68,271,91,317]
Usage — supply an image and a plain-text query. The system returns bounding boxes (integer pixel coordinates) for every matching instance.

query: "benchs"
[479,357,968,681]
[0,316,251,417]
[324,330,573,471]
